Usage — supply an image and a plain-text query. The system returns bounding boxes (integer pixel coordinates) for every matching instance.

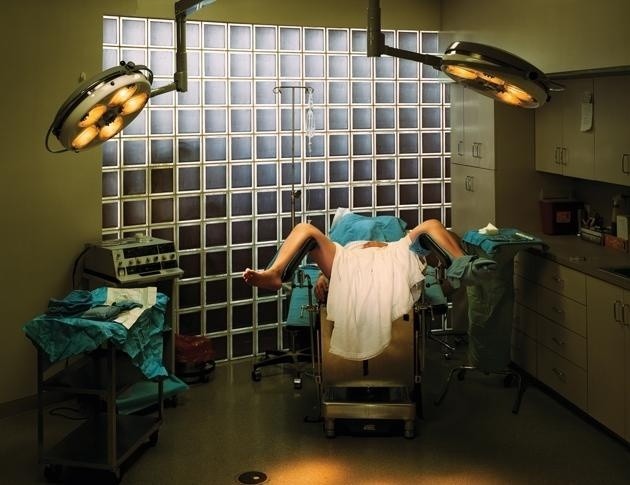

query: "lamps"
[367,0,564,111]
[45,0,217,154]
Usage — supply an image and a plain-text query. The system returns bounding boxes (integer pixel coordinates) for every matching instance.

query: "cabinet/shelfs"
[586,275,630,442]
[450,82,535,170]
[511,251,587,413]
[535,77,594,180]
[594,75,630,186]
[450,164,562,239]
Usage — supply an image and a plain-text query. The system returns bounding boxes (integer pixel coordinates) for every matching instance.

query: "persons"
[243,218,468,305]
